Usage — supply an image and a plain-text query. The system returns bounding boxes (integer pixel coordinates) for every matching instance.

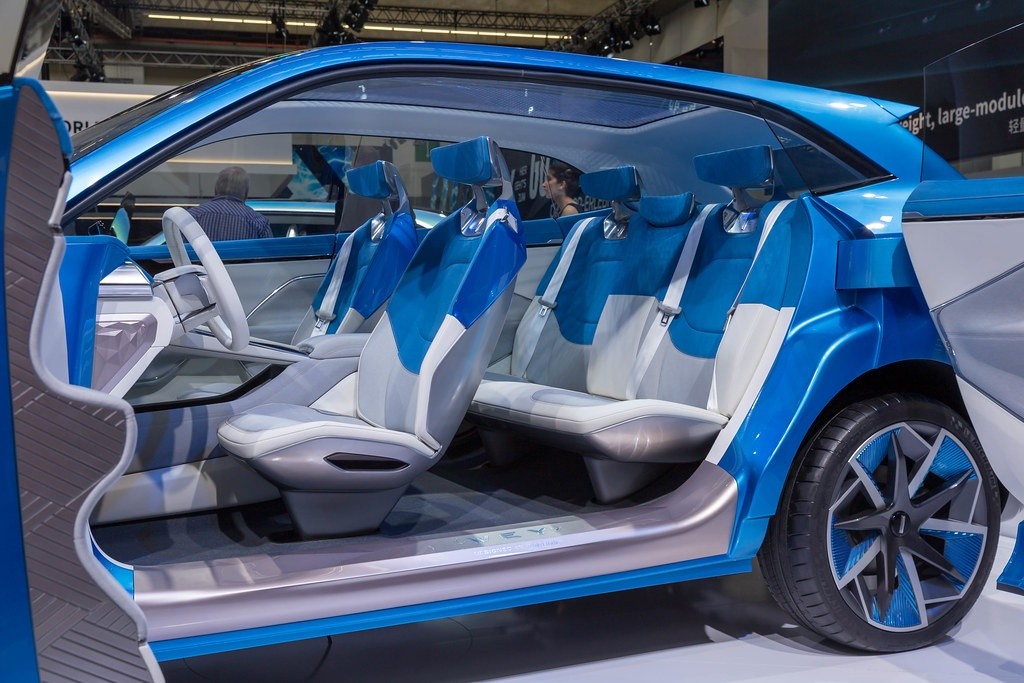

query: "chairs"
[215,134,527,540]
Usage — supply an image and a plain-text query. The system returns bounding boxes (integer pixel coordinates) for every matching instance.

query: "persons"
[543,163,583,217]
[181,167,273,244]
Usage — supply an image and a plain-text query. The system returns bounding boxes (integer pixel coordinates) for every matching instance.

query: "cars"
[0,37,1024,683]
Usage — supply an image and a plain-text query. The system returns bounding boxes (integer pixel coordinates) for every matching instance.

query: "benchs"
[467,144,811,503]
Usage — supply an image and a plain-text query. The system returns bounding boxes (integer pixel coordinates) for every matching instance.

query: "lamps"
[577,12,662,56]
[312,0,378,48]
[270,10,289,38]
[693,0,710,8]
